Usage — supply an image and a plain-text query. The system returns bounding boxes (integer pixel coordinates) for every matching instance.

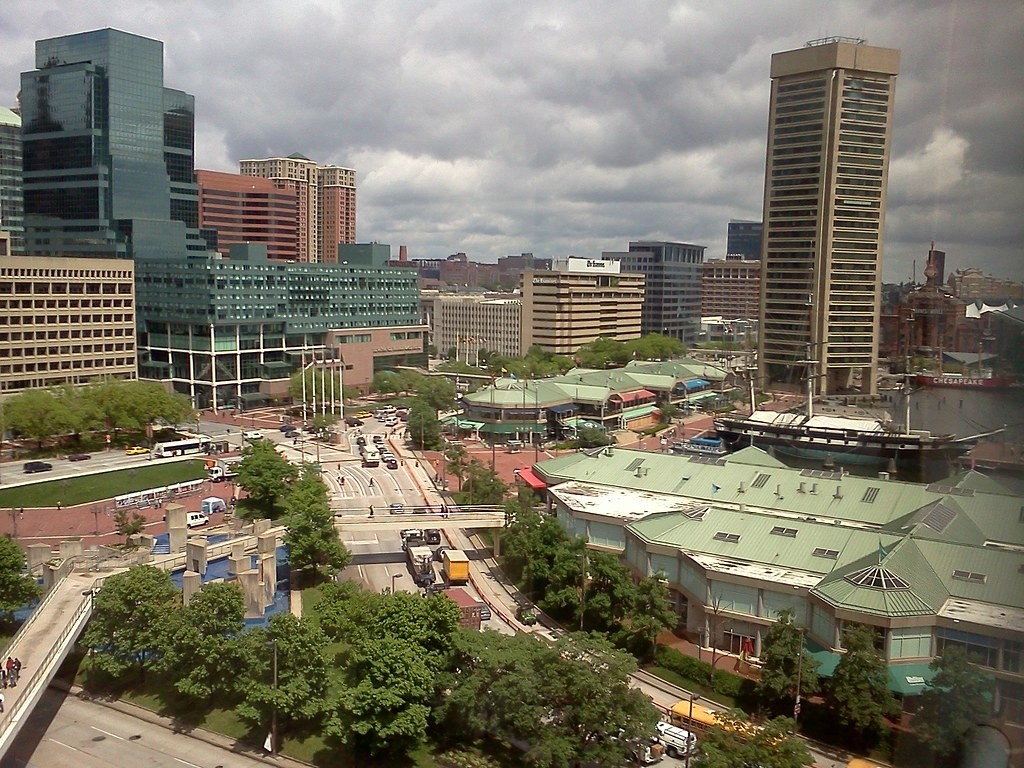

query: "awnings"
[560,418,608,430]
[687,393,718,403]
[621,406,659,419]
[442,417,545,435]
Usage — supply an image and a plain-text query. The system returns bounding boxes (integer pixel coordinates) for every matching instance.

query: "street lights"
[392,573,403,593]
[685,692,701,767]
[793,627,804,738]
[264,640,279,755]
[576,553,585,630]
[82,589,94,657]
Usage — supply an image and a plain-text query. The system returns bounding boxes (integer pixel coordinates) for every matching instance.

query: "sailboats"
[710,239,1009,473]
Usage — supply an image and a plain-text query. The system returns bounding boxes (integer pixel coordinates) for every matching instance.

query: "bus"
[669,700,787,754]
[153,436,211,457]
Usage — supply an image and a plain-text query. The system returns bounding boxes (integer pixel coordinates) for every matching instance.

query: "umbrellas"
[743,637,753,659]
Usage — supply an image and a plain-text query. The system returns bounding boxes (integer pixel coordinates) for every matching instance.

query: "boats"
[677,437,728,455]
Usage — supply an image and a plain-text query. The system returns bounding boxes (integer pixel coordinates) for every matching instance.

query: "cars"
[68,453,91,462]
[343,400,414,469]
[515,604,537,627]
[125,446,150,456]
[390,503,404,514]
[473,602,491,620]
[281,424,318,440]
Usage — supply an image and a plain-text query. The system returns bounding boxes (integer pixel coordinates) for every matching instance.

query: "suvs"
[23,461,53,473]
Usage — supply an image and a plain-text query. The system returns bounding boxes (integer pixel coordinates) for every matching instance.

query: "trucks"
[649,721,697,759]
[400,527,484,631]
[208,455,250,483]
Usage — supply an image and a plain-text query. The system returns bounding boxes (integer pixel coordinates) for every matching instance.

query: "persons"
[0,657,21,713]
[57,500,61,510]
[369,477,375,487]
[337,464,341,470]
[445,506,449,519]
[158,499,162,508]
[337,477,345,486]
[19,507,24,520]
[369,505,375,518]
[154,499,159,510]
[384,428,409,439]
[440,503,445,518]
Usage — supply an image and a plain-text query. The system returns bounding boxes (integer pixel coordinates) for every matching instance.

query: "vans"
[186,511,209,529]
[244,429,265,441]
